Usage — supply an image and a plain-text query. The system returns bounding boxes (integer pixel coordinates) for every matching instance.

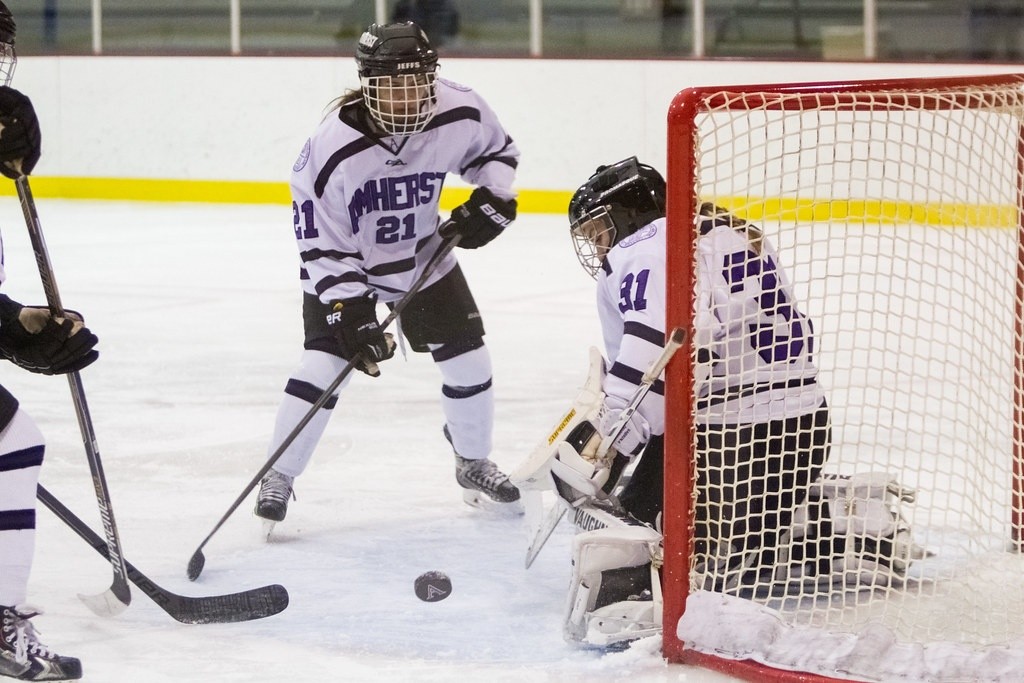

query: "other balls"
[414,569,451,604]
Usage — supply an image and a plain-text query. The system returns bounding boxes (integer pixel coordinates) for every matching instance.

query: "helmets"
[570,155,666,282]
[0,3,18,85]
[354,21,438,134]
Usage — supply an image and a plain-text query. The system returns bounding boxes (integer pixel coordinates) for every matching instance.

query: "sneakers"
[443,422,527,515]
[255,469,295,545]
[1,603,82,681]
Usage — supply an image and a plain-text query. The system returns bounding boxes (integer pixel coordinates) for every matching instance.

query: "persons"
[251,18,526,523]
[547,153,834,597]
[0,1,102,683]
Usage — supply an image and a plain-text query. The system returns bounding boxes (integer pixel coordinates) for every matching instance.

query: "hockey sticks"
[14,171,136,623]
[32,477,292,626]
[183,231,460,587]
[520,324,689,574]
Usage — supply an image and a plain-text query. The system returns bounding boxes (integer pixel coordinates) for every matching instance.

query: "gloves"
[0,84,42,179]
[438,187,518,249]
[326,296,397,379]
[1,294,101,374]
